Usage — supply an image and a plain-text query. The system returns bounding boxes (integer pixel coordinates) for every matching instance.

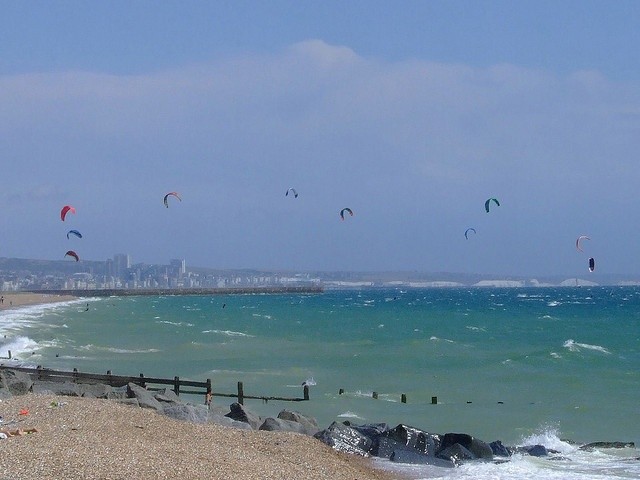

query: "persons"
[205,387,212,406]
